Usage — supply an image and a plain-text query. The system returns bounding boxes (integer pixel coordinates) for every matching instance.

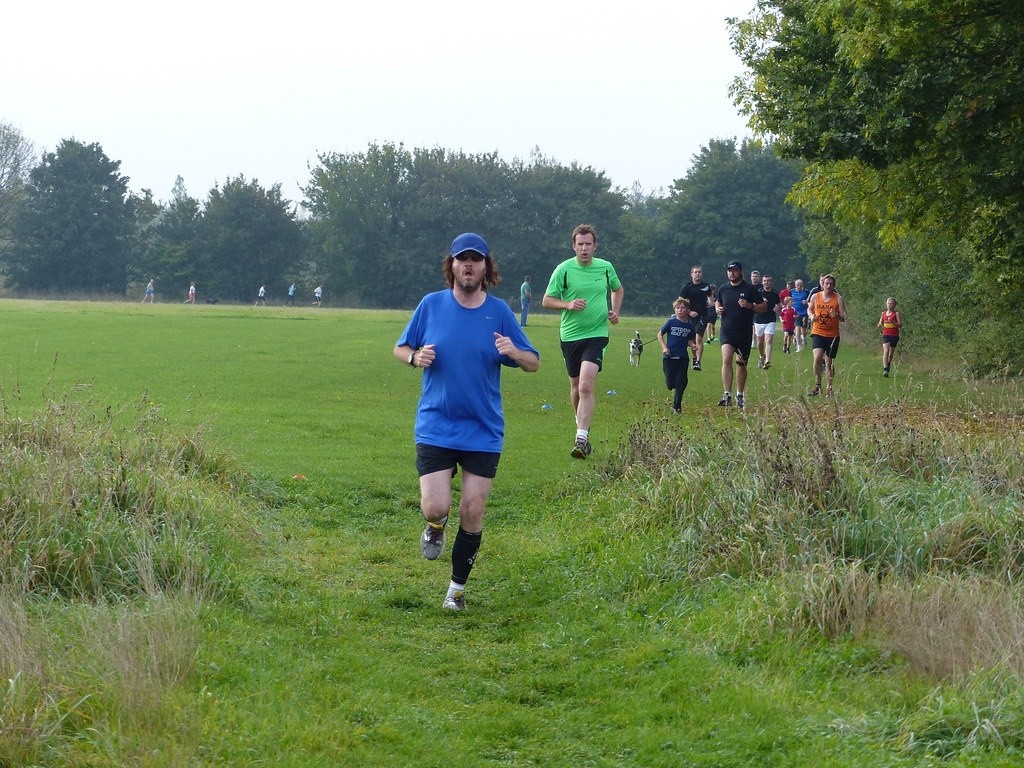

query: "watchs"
[750,304,754,310]
[408,352,418,369]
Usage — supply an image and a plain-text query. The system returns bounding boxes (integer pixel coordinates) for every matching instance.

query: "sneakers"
[735,395,744,407]
[444,591,466,612]
[717,393,732,406]
[419,507,451,561]
[572,441,591,459]
[692,358,701,370]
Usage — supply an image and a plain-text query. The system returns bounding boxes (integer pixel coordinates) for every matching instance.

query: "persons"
[309,282,323,308]
[542,224,624,460]
[141,278,155,303]
[394,233,540,613]
[657,299,698,415]
[878,296,902,378]
[715,259,767,409]
[254,284,267,307]
[282,281,296,308]
[806,273,848,398]
[521,276,532,327]
[754,274,782,370]
[778,279,810,354]
[184,281,196,304]
[679,265,718,371]
[749,270,763,349]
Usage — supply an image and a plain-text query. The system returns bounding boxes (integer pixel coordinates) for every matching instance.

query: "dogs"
[202,297,220,305]
[629,331,644,367]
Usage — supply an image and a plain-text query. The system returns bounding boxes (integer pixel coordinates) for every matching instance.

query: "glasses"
[456,254,484,262]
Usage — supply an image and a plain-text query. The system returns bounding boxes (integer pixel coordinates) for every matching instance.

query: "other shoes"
[882,369,888,376]
[796,348,801,353]
[826,389,832,397]
[808,387,822,396]
[763,362,771,369]
[783,345,790,353]
[751,342,755,349]
[757,356,764,368]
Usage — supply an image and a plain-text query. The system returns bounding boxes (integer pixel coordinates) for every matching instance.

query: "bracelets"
[836,313,840,318]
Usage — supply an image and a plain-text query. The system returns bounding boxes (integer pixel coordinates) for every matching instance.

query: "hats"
[450,232,489,258]
[726,261,741,270]
